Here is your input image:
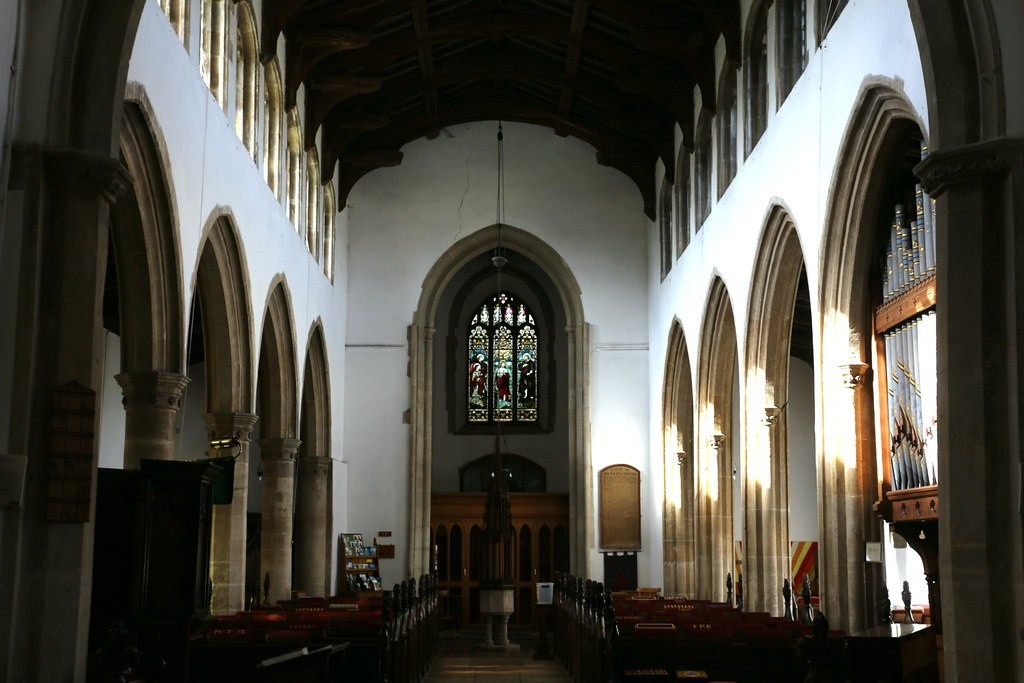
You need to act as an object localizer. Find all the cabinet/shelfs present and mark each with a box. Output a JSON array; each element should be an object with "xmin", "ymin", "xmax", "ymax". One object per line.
[{"xmin": 340, "ymin": 533, "xmax": 382, "ymax": 599}]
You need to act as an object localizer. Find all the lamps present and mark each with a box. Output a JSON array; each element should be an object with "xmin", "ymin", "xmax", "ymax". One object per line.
[
  {"xmin": 465, "ymin": 116, "xmax": 540, "ymax": 422},
  {"xmin": 207, "ymin": 430, "xmax": 242, "ymax": 458}
]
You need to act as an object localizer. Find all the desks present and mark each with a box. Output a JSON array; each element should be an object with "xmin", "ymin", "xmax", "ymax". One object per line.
[{"xmin": 846, "ymin": 623, "xmax": 938, "ymax": 683}]
